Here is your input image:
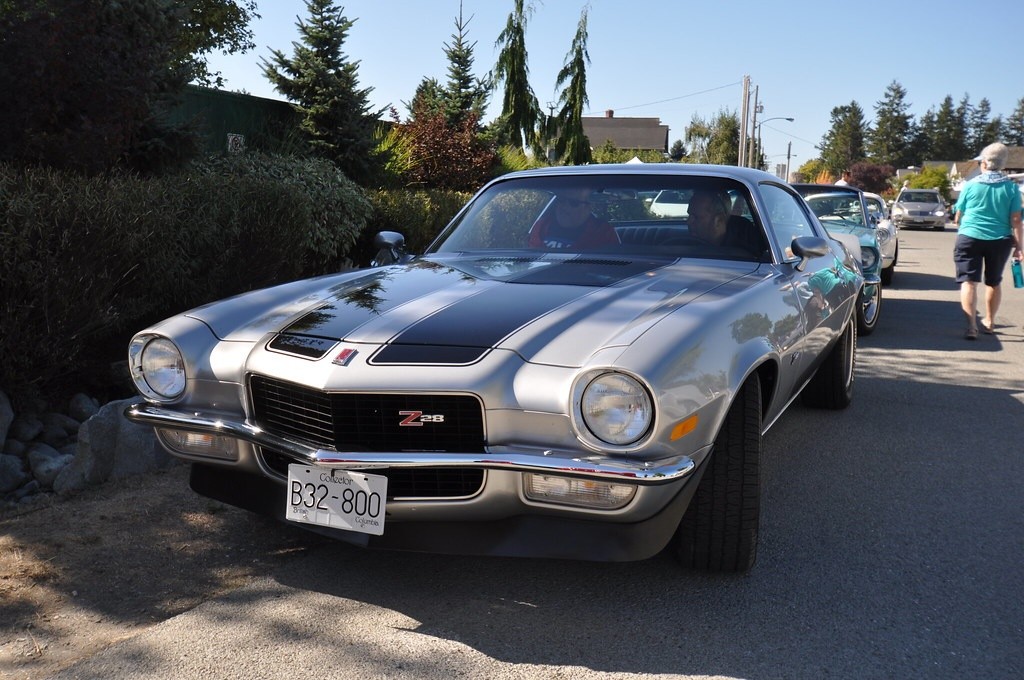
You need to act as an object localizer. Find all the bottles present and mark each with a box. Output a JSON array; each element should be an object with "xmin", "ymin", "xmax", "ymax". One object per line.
[{"xmin": 1011, "ymin": 256, "xmax": 1024, "ymax": 288}]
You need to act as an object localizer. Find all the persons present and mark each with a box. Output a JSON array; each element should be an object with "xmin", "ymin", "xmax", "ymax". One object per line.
[
  {"xmin": 954, "ymin": 142, "xmax": 1024, "ymax": 338},
  {"xmin": 901, "ymin": 179, "xmax": 911, "ymax": 191},
  {"xmin": 686, "ymin": 188, "xmax": 755, "ymax": 253},
  {"xmin": 526, "ymin": 184, "xmax": 622, "ymax": 250},
  {"xmin": 834, "ymin": 171, "xmax": 852, "ymax": 186},
  {"xmin": 803, "ymin": 256, "xmax": 866, "ymax": 319}
]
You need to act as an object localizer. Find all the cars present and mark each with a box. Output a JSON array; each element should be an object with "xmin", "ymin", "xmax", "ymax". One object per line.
[
  {"xmin": 726, "ymin": 182, "xmax": 884, "ymax": 337},
  {"xmin": 646, "ymin": 190, "xmax": 691, "ymax": 221},
  {"xmin": 121, "ymin": 161, "xmax": 870, "ymax": 579},
  {"xmin": 804, "ymin": 191, "xmax": 900, "ymax": 287},
  {"xmin": 888, "ymin": 188, "xmax": 950, "ymax": 231}
]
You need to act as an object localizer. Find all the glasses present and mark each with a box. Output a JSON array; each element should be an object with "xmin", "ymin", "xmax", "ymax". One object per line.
[{"xmin": 556, "ymin": 194, "xmax": 592, "ymax": 208}]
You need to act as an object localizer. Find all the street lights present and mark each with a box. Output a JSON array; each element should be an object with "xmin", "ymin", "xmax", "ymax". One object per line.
[{"xmin": 748, "ymin": 84, "xmax": 795, "ymax": 170}]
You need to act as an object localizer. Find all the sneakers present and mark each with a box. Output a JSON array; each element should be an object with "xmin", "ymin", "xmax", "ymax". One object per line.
[
  {"xmin": 965, "ymin": 327, "xmax": 979, "ymax": 339},
  {"xmin": 978, "ymin": 319, "xmax": 993, "ymax": 334}
]
